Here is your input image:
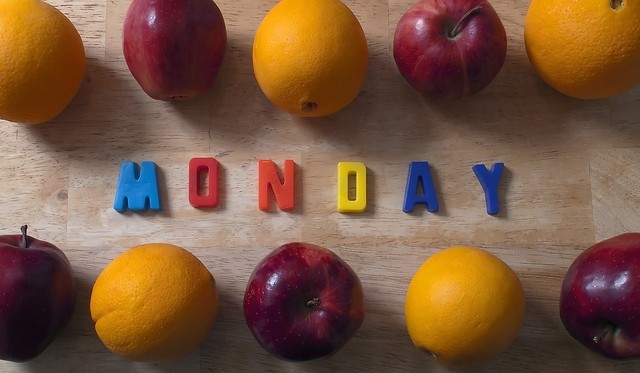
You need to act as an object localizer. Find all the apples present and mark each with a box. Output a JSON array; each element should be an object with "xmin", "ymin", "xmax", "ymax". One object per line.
[
  {"xmin": 242, "ymin": 241, "xmax": 365, "ymax": 361},
  {"xmin": 122, "ymin": 1, "xmax": 226, "ymax": 103},
  {"xmin": 559, "ymin": 232, "xmax": 640, "ymax": 361},
  {"xmin": 1, "ymin": 225, "xmax": 76, "ymax": 363},
  {"xmin": 393, "ymin": 0, "xmax": 508, "ymax": 100}
]
